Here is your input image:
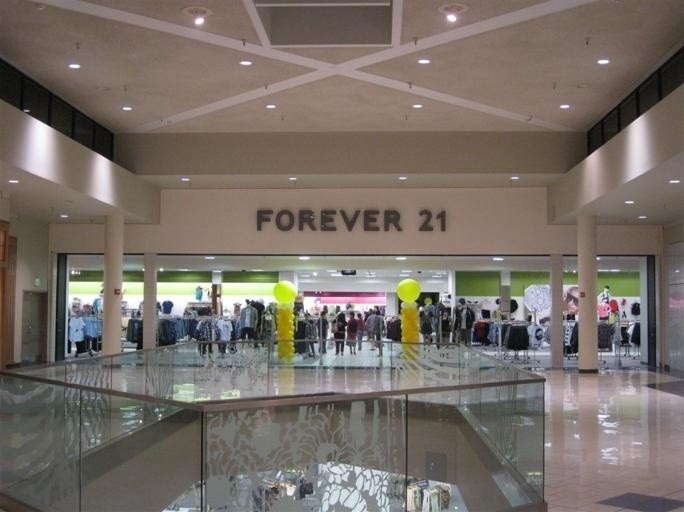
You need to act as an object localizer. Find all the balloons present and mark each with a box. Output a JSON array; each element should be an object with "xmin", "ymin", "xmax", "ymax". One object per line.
[
  {"xmin": 397, "ymin": 278, "xmax": 421, "ymax": 357},
  {"xmin": 273, "ymin": 279, "xmax": 297, "ymax": 360}
]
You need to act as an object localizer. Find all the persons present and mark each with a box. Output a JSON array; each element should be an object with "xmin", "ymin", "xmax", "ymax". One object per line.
[
  {"xmin": 238, "ymin": 298, "xmax": 260, "ymax": 351},
  {"xmin": 416, "ymin": 294, "xmax": 476, "ymax": 352},
  {"xmin": 563, "ymin": 286, "xmax": 580, "ymax": 315},
  {"xmin": 596, "ymin": 298, "xmax": 612, "ymax": 322},
  {"xmin": 597, "ymin": 285, "xmax": 613, "ymax": 304},
  {"xmin": 294, "ymin": 304, "xmax": 384, "ymax": 359}
]
